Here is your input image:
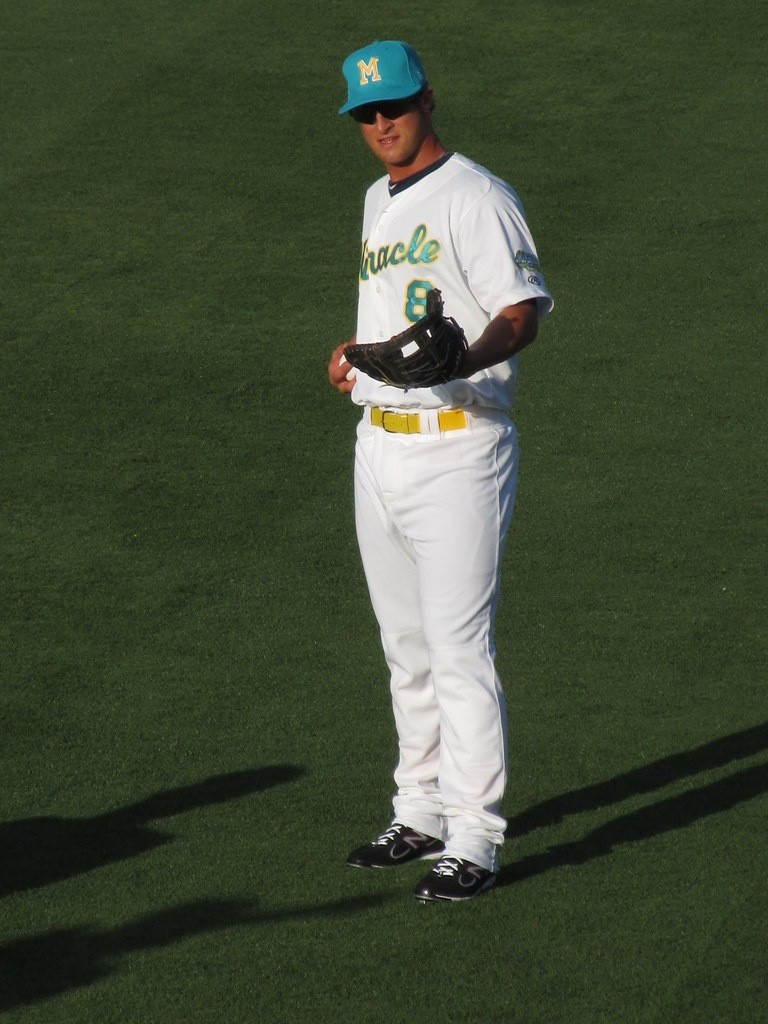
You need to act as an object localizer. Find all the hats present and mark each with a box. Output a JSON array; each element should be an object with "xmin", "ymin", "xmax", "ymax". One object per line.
[{"xmin": 338, "ymin": 39, "xmax": 427, "ymax": 115}]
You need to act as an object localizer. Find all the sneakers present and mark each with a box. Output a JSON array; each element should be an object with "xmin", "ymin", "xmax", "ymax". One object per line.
[
  {"xmin": 344, "ymin": 818, "xmax": 441, "ymax": 870},
  {"xmin": 414, "ymin": 845, "xmax": 498, "ymax": 900}
]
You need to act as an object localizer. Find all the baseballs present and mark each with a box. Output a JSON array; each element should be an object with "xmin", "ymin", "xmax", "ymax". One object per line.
[{"xmin": 337, "ymin": 353, "xmax": 358, "ymax": 383}]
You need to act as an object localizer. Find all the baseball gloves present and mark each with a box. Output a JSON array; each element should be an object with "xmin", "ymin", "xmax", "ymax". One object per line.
[{"xmin": 342, "ymin": 288, "xmax": 471, "ymax": 394}]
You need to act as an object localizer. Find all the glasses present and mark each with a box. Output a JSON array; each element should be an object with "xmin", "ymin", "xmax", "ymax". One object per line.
[{"xmin": 349, "ymin": 97, "xmax": 420, "ymax": 124}]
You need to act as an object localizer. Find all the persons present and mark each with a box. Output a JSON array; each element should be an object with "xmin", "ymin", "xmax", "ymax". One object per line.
[{"xmin": 327, "ymin": 39, "xmax": 556, "ymax": 907}]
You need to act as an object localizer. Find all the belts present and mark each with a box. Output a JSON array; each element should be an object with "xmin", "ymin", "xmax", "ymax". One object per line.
[{"xmin": 372, "ymin": 407, "xmax": 466, "ymax": 432}]
